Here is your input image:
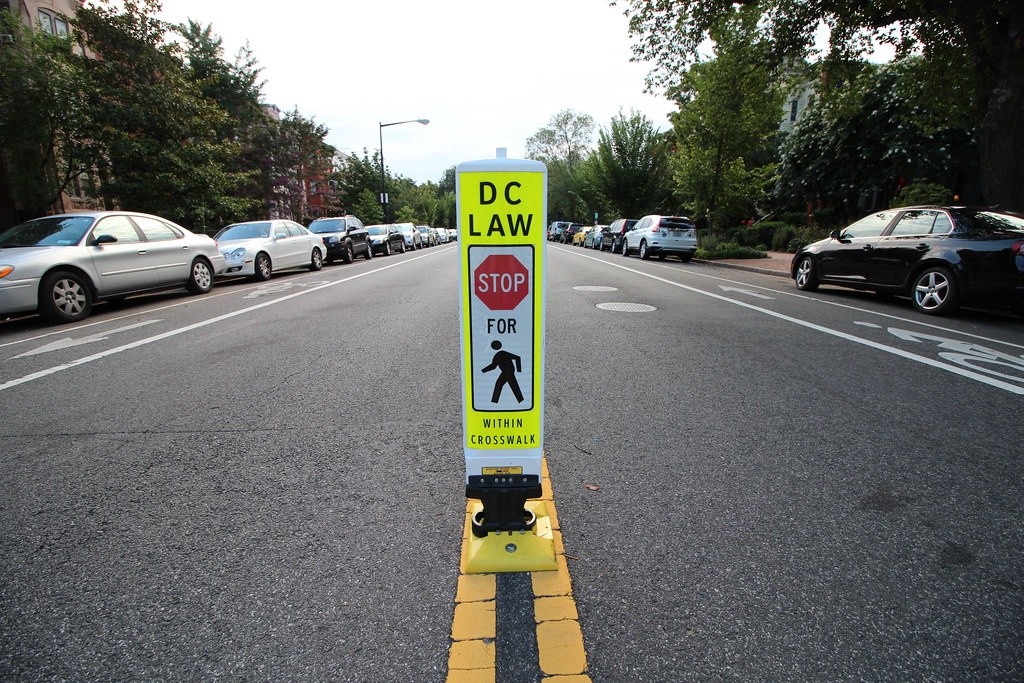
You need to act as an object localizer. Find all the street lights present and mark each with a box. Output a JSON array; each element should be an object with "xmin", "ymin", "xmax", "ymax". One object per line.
[{"xmin": 378, "ymin": 118, "xmax": 430, "ymax": 223}]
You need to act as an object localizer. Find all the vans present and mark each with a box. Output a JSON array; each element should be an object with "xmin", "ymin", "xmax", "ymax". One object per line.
[{"xmin": 550, "ymin": 220, "xmax": 571, "ymax": 240}]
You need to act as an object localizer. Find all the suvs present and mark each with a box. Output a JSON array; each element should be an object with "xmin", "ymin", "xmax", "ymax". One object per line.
[
  {"xmin": 600, "ymin": 219, "xmax": 639, "ymax": 254},
  {"xmin": 621, "ymin": 214, "xmax": 699, "ymax": 263},
  {"xmin": 307, "ymin": 215, "xmax": 374, "ymax": 262},
  {"xmin": 559, "ymin": 222, "xmax": 580, "ymax": 243}
]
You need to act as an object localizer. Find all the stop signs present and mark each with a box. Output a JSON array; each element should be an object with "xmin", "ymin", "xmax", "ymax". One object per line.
[{"xmin": 459, "ymin": 170, "xmax": 540, "ymax": 478}]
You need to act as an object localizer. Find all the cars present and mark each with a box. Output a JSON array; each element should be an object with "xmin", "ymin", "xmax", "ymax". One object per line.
[
  {"xmin": 584, "ymin": 225, "xmax": 609, "ymax": 249},
  {"xmin": 790, "ymin": 204, "xmax": 1024, "ymax": 323},
  {"xmin": 547, "ymin": 225, "xmax": 551, "ymax": 238},
  {"xmin": 572, "ymin": 226, "xmax": 593, "ymax": 247},
  {"xmin": 1, "ymin": 208, "xmax": 226, "ymax": 323},
  {"xmin": 365, "ymin": 225, "xmax": 406, "ymax": 254},
  {"xmin": 213, "ymin": 218, "xmax": 328, "ymax": 280},
  {"xmin": 393, "ymin": 222, "xmax": 458, "ymax": 251}
]
